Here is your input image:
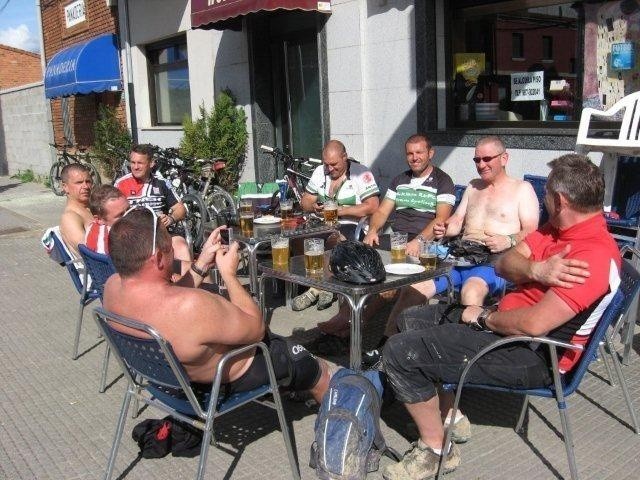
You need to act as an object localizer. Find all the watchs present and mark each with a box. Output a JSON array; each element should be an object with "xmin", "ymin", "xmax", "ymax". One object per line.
[
  {"xmin": 477, "ymin": 309, "xmax": 493, "ymax": 334},
  {"xmin": 508, "ymin": 235, "xmax": 517, "ymax": 249}
]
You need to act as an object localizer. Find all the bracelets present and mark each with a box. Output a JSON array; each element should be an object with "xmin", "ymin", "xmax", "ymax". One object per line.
[
  {"xmin": 414, "ymin": 235, "xmax": 426, "ymax": 243},
  {"xmin": 189, "ymin": 263, "xmax": 209, "ymax": 279},
  {"xmin": 170, "ymin": 216, "xmax": 177, "ymax": 222}
]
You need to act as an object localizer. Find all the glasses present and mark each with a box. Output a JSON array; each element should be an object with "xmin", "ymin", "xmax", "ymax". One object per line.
[
  {"xmin": 116, "ymin": 202, "xmax": 161, "ymax": 257},
  {"xmin": 472, "ymin": 152, "xmax": 505, "ymax": 163}
]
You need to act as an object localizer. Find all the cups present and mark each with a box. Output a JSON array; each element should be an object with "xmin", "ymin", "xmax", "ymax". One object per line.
[
  {"xmin": 271, "ymin": 237, "xmax": 289, "ymax": 265},
  {"xmin": 216, "ymin": 200, "xmax": 339, "ymax": 233},
  {"xmin": 419, "ymin": 241, "xmax": 437, "ymax": 272},
  {"xmin": 390, "ymin": 231, "xmax": 408, "ymax": 260},
  {"xmin": 304, "ymin": 238, "xmax": 323, "ymax": 273}
]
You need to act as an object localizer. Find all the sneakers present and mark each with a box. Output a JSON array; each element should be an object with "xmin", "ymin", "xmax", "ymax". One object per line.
[
  {"xmin": 380, "ymin": 434, "xmax": 463, "ymax": 479},
  {"xmin": 291, "ymin": 290, "xmax": 316, "ymax": 311},
  {"xmin": 316, "ymin": 290, "xmax": 335, "ymax": 311},
  {"xmin": 443, "ymin": 415, "xmax": 475, "ymax": 444}
]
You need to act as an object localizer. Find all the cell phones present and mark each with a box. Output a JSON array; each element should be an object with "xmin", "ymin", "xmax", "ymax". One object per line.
[{"xmin": 220, "ymin": 228, "xmax": 233, "ymax": 253}]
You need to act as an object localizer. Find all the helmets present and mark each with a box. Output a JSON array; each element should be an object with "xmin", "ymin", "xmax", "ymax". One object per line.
[{"xmin": 328, "ymin": 239, "xmax": 388, "ymax": 286}]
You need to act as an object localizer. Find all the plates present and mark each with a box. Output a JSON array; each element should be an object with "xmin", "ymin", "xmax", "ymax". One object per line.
[{"xmin": 383, "ymin": 263, "xmax": 424, "ymax": 276}]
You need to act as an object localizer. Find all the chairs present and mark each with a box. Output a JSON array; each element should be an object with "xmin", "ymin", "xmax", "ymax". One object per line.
[{"xmin": 50, "ymin": 194, "xmax": 640, "ymax": 480}]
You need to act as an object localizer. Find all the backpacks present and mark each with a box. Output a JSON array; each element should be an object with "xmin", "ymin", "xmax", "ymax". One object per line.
[{"xmin": 307, "ymin": 366, "xmax": 391, "ymax": 480}]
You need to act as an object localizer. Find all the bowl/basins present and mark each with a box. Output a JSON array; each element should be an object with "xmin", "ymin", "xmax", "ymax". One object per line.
[{"xmin": 477, "ymin": 103, "xmax": 499, "ymax": 120}]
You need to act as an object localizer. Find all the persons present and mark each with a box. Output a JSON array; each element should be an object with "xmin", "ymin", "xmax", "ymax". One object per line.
[
  {"xmin": 60, "ymin": 164, "xmax": 191, "ymax": 261},
  {"xmin": 103, "ymin": 206, "xmax": 330, "ymax": 407},
  {"xmin": 84, "ymin": 185, "xmax": 193, "ymax": 282},
  {"xmin": 317, "ymin": 134, "xmax": 456, "ymax": 339},
  {"xmin": 382, "ymin": 153, "xmax": 621, "ymax": 479},
  {"xmin": 291, "ymin": 140, "xmax": 381, "ymax": 311},
  {"xmin": 113, "ymin": 145, "xmax": 187, "ymax": 228},
  {"xmin": 377, "ymin": 135, "xmax": 540, "ymax": 358}
]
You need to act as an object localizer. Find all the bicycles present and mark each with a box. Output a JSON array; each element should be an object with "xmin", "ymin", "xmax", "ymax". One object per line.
[
  {"xmin": 103, "ymin": 141, "xmax": 237, "ymax": 252},
  {"xmin": 259, "ymin": 142, "xmax": 325, "ymax": 213},
  {"xmin": 47, "ymin": 141, "xmax": 102, "ymax": 194}
]
